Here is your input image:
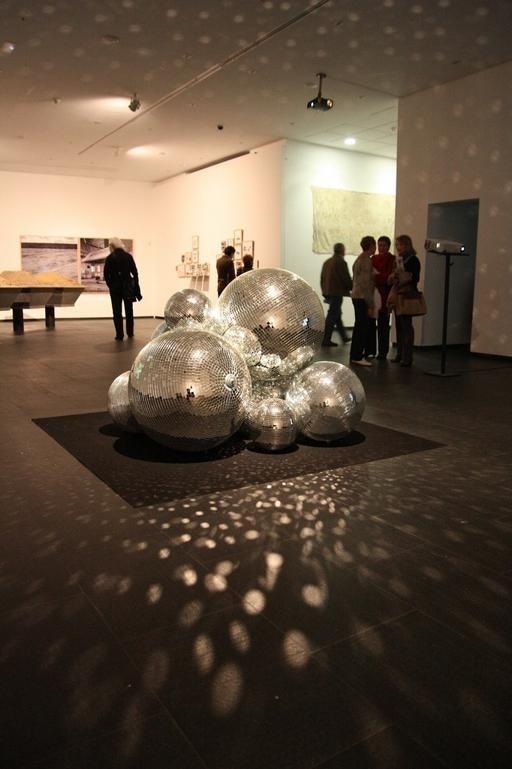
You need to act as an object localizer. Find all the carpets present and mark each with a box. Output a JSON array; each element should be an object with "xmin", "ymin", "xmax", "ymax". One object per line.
[{"xmin": 32, "ymin": 411, "xmax": 447, "ymax": 508}]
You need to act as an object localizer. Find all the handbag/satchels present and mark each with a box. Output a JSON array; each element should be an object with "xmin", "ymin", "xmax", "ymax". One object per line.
[{"xmin": 395, "ymin": 291, "xmax": 427, "ymax": 316}]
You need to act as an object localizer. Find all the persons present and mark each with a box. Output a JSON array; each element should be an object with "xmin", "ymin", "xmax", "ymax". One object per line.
[
  {"xmin": 237, "ymin": 254, "xmax": 254, "ymax": 278},
  {"xmin": 386, "ymin": 234, "xmax": 421, "ymax": 367},
  {"xmin": 349, "ymin": 236, "xmax": 376, "ymax": 366},
  {"xmin": 320, "ymin": 243, "xmax": 353, "ymax": 347},
  {"xmin": 103, "ymin": 237, "xmax": 142, "ymax": 340},
  {"xmin": 216, "ymin": 246, "xmax": 235, "ymax": 297},
  {"xmin": 370, "ymin": 236, "xmax": 396, "ymax": 359}
]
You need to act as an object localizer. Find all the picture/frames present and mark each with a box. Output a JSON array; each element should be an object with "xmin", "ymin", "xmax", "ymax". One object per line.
[
  {"xmin": 216, "ymin": 229, "xmax": 259, "ymax": 274},
  {"xmin": 175, "ymin": 235, "xmax": 210, "ymax": 277}
]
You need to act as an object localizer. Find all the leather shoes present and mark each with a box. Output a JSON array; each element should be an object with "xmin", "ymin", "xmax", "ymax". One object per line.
[
  {"xmin": 322, "ymin": 341, "xmax": 337, "ymax": 346},
  {"xmin": 351, "ymin": 357, "xmax": 372, "ymax": 366},
  {"xmin": 400, "ymin": 359, "xmax": 411, "ymax": 367},
  {"xmin": 391, "ymin": 358, "xmax": 400, "ymax": 362}
]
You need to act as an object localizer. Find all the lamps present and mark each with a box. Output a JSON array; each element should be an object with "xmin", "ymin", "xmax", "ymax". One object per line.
[{"xmin": 128, "ymin": 94, "xmax": 140, "ymax": 112}]
